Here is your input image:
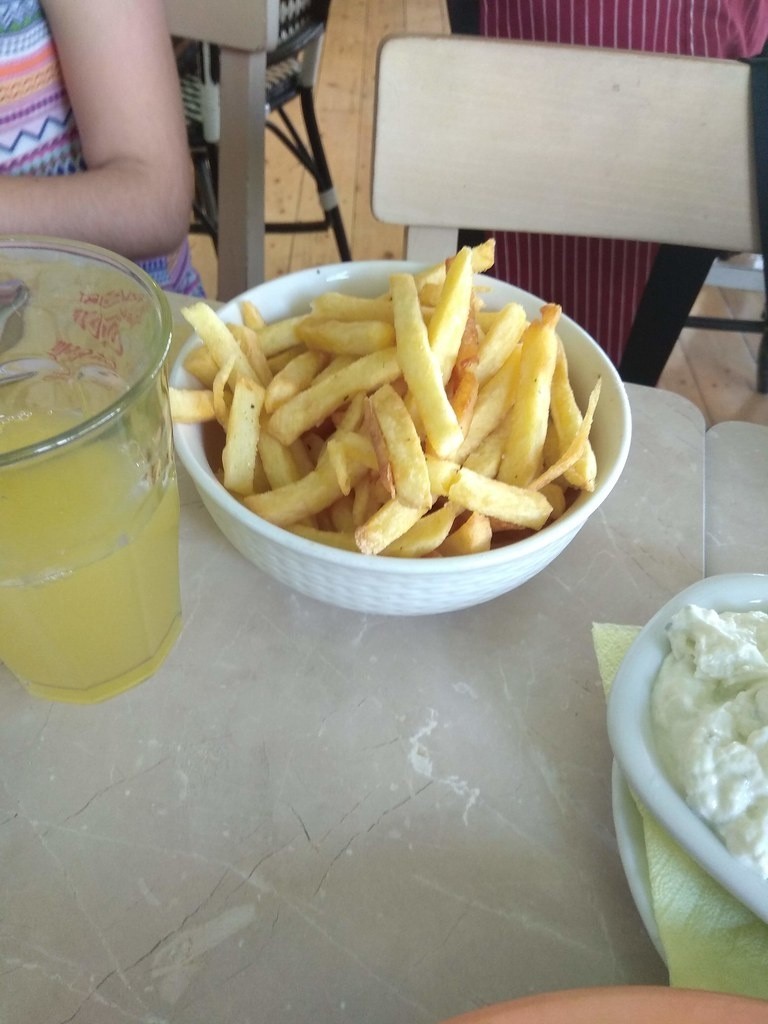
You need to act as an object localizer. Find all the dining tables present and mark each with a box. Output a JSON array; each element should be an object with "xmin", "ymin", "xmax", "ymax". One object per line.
[{"xmin": 0, "ymin": 384, "xmax": 768, "ymax": 1024}]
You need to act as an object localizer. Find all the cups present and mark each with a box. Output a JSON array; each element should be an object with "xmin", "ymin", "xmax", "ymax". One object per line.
[{"xmin": 0, "ymin": 233, "xmax": 181, "ymax": 705}]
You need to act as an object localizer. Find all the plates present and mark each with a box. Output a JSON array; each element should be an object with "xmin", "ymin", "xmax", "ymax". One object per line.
[{"xmin": 607, "ymin": 572, "xmax": 768, "ymax": 965}]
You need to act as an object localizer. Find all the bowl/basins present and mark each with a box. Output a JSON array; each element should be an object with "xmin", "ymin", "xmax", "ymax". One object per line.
[{"xmin": 168, "ymin": 259, "xmax": 633, "ymax": 616}]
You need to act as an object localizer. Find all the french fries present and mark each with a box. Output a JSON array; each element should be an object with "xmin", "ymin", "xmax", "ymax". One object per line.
[{"xmin": 167, "ymin": 240, "xmax": 598, "ymax": 558}]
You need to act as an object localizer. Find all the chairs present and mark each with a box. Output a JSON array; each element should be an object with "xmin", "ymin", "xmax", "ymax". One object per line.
[{"xmin": 163, "ymin": 0, "xmax": 768, "ymax": 395}]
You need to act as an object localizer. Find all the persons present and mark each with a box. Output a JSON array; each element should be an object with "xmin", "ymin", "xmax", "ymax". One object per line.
[
  {"xmin": 446, "ymin": 0, "xmax": 767, "ymax": 387},
  {"xmin": 0, "ymin": 0, "xmax": 206, "ymax": 301}
]
